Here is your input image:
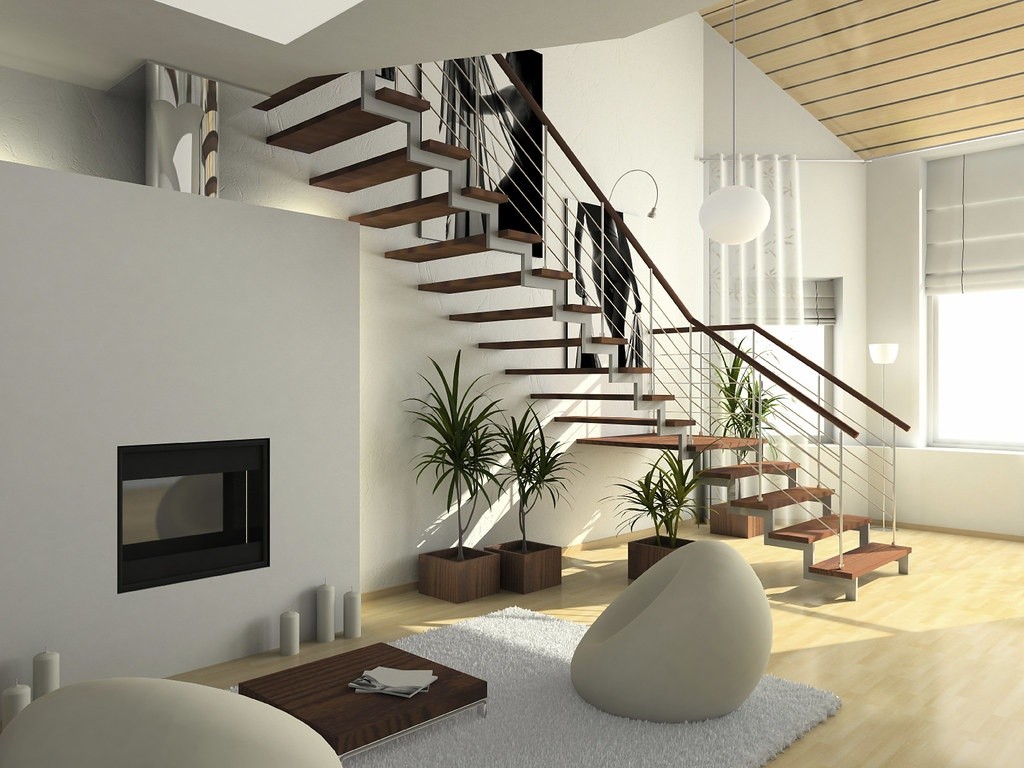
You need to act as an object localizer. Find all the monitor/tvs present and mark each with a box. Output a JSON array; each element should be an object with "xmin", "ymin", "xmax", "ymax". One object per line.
[{"xmin": 120, "ymin": 470, "xmax": 249, "ymax": 561}]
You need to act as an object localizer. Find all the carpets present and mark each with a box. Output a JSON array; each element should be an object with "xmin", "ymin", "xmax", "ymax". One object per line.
[{"xmin": 225, "ymin": 606, "xmax": 841, "ymax": 768}]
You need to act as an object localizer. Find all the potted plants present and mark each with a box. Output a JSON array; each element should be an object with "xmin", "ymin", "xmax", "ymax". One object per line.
[
  {"xmin": 409, "ymin": 351, "xmax": 500, "ymax": 604},
  {"xmin": 709, "ymin": 333, "xmax": 787, "ymax": 540},
  {"xmin": 482, "ymin": 396, "xmax": 586, "ymax": 594},
  {"xmin": 611, "ymin": 439, "xmax": 718, "ymax": 581}
]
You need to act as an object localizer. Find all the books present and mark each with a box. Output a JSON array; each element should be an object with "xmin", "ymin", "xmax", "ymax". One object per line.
[{"xmin": 348, "ymin": 666, "xmax": 438, "ymax": 698}]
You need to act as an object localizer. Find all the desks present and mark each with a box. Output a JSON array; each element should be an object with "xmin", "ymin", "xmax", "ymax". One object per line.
[{"xmin": 238, "ymin": 641, "xmax": 488, "ymax": 759}]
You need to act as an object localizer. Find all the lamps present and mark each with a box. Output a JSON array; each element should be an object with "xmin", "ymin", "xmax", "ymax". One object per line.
[
  {"xmin": 608, "ymin": 168, "xmax": 660, "ymax": 218},
  {"xmin": 698, "ymin": 0, "xmax": 770, "ymax": 247},
  {"xmin": 869, "ymin": 342, "xmax": 899, "ymax": 530}
]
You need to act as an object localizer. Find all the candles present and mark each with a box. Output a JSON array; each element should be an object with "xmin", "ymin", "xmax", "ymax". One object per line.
[
  {"xmin": 316, "ymin": 578, "xmax": 335, "ymax": 643},
  {"xmin": 33, "ymin": 646, "xmax": 60, "ymax": 702},
  {"xmin": 343, "ymin": 586, "xmax": 361, "ymax": 639},
  {"xmin": 3, "ymin": 677, "xmax": 33, "ymax": 733},
  {"xmin": 280, "ymin": 609, "xmax": 300, "ymax": 658}
]
